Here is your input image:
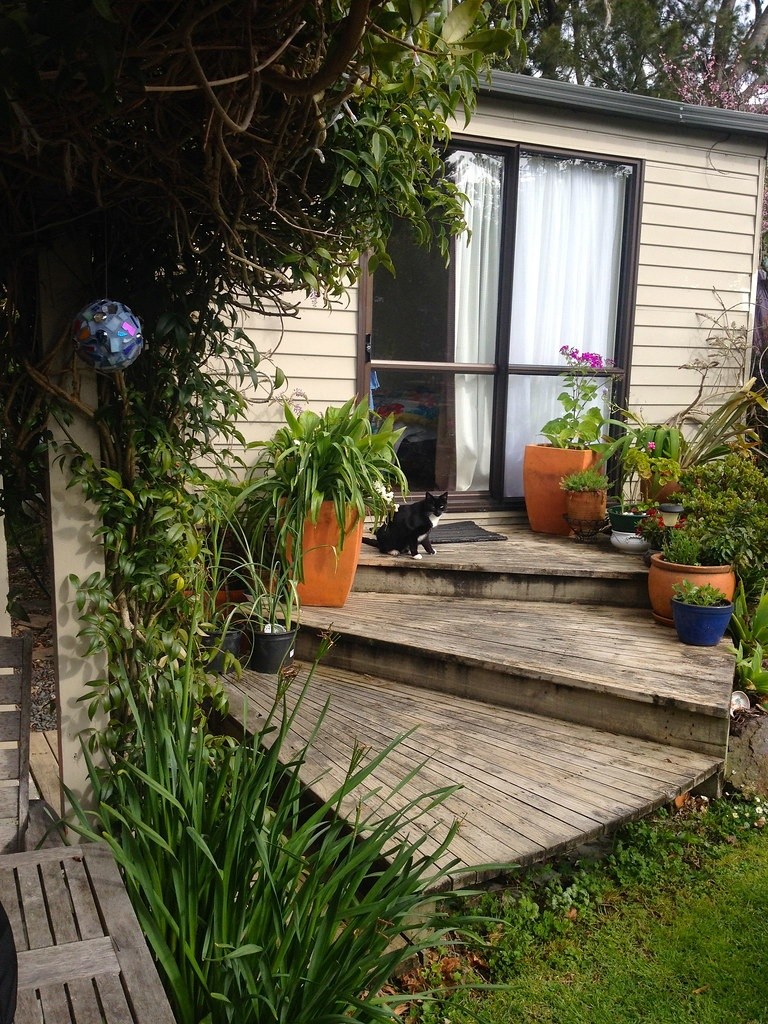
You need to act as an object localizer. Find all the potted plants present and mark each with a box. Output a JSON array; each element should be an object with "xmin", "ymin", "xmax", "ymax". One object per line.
[
  {"xmin": 201, "ymin": 488, "xmax": 246, "ymax": 676},
  {"xmin": 668, "ymin": 576, "xmax": 734, "ymax": 646},
  {"xmin": 559, "ymin": 464, "xmax": 619, "ymax": 522},
  {"xmin": 204, "ymin": 488, "xmax": 340, "ymax": 675},
  {"xmin": 645, "ymin": 527, "xmax": 738, "ymax": 629},
  {"xmin": 635, "ymin": 426, "xmax": 694, "ymax": 503},
  {"xmin": 249, "ymin": 388, "xmax": 411, "ymax": 610}
]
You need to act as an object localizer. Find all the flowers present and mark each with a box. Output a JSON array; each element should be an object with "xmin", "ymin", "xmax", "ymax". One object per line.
[
  {"xmin": 534, "ymin": 344, "xmax": 627, "ymax": 450},
  {"xmin": 608, "ymin": 438, "xmax": 680, "ymax": 513}
]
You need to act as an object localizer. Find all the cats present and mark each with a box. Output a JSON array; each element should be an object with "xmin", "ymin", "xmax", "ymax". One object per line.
[{"xmin": 361, "ymin": 491, "xmax": 449, "ymax": 561}]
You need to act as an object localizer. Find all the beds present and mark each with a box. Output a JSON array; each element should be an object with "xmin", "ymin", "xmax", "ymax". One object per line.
[{"xmin": 377, "ymin": 384, "xmax": 442, "ymax": 486}]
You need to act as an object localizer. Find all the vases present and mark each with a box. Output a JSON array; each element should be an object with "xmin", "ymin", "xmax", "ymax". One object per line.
[
  {"xmin": 524, "ymin": 442, "xmax": 599, "ymax": 535},
  {"xmin": 606, "ymin": 503, "xmax": 655, "ymax": 556}
]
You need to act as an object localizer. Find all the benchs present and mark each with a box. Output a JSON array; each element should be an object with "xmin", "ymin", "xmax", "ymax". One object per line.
[{"xmin": 0, "ymin": 838, "xmax": 177, "ymax": 1024}]
